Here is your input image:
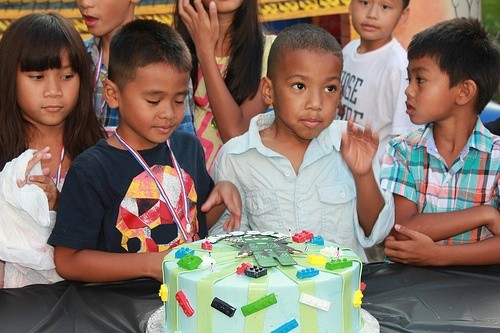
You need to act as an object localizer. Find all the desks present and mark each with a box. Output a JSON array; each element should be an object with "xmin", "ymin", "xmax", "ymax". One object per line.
[{"xmin": 0, "ymin": 262, "xmax": 500, "ymax": 333}]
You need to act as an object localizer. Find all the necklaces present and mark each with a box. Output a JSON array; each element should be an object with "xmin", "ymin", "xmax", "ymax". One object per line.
[
  {"xmin": 55, "ymin": 144, "xmax": 65, "ymax": 190},
  {"xmin": 92, "ymin": 38, "xmax": 107, "ymax": 125},
  {"xmin": 113, "ymin": 128, "xmax": 191, "ymax": 241}
]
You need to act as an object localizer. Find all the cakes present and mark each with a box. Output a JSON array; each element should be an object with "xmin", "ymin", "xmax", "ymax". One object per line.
[{"xmin": 158, "ymin": 230, "xmax": 366, "ymax": 333}]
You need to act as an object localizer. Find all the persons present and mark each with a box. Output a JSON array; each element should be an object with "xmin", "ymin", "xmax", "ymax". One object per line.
[
  {"xmin": 174, "ymin": 1, "xmax": 281, "ymax": 175},
  {"xmin": 47, "ymin": 19, "xmax": 242, "ymax": 283},
  {"xmin": 336, "ymin": 0, "xmax": 426, "ymax": 167},
  {"xmin": 0, "ymin": 12, "xmax": 108, "ymax": 288},
  {"xmin": 75, "ymin": 0, "xmax": 197, "ymax": 138},
  {"xmin": 207, "ymin": 24, "xmax": 395, "ymax": 264},
  {"xmin": 379, "ymin": 17, "xmax": 500, "ymax": 266}
]
